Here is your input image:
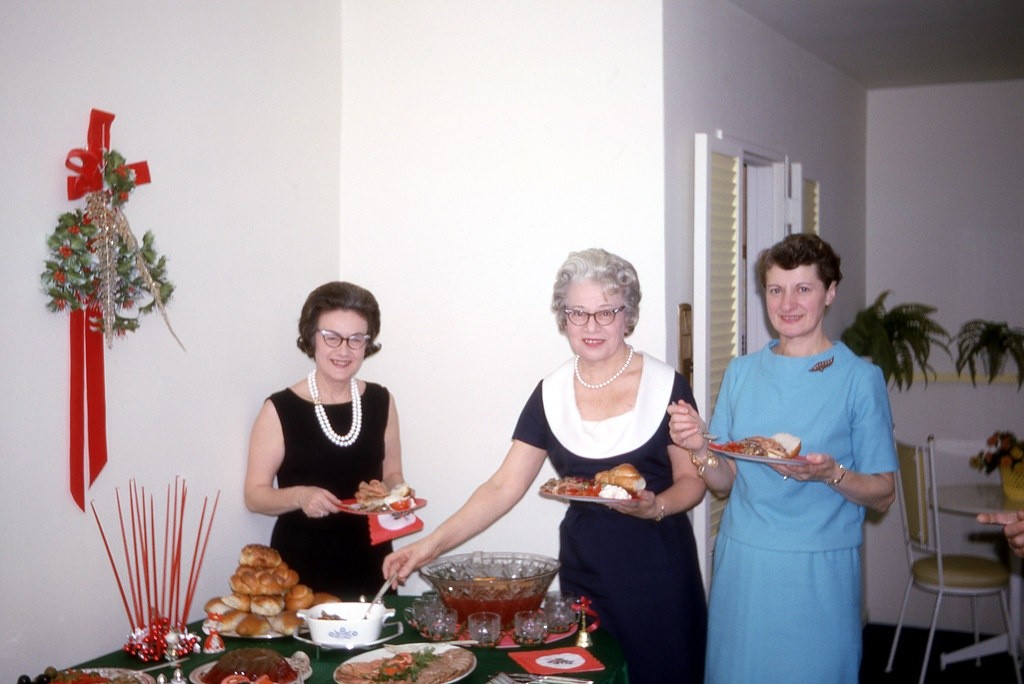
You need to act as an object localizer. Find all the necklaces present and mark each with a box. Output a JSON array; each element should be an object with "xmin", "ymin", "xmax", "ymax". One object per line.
[
  {"xmin": 308, "ymin": 370, "xmax": 362, "ymax": 447},
  {"xmin": 575, "ymin": 346, "xmax": 634, "ymax": 389}
]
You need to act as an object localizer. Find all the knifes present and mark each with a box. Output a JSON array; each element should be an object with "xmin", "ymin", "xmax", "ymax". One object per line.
[{"xmin": 488, "ymin": 672, "xmax": 594, "ymax": 684}]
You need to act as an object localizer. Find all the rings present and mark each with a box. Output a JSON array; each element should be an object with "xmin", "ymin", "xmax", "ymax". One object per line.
[{"xmin": 784, "ymin": 474, "xmax": 789, "ymax": 481}]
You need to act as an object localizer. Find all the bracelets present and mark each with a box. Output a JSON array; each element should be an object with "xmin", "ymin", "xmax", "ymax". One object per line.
[
  {"xmin": 688, "ymin": 432, "xmax": 719, "ymax": 477},
  {"xmin": 825, "ymin": 463, "xmax": 847, "ymax": 487},
  {"xmin": 654, "ymin": 497, "xmax": 666, "ymax": 521}
]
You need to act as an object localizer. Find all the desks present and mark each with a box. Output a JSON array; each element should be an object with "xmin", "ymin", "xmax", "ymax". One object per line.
[
  {"xmin": 76, "ymin": 594, "xmax": 629, "ymax": 684},
  {"xmin": 927, "ymin": 483, "xmax": 1024, "ymax": 674}
]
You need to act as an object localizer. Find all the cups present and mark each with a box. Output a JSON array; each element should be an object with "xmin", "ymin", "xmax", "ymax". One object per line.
[
  {"xmin": 544, "ymin": 591, "xmax": 581, "ymax": 633},
  {"xmin": 403, "ymin": 598, "xmax": 444, "ymax": 629},
  {"xmin": 425, "ymin": 608, "xmax": 457, "ymax": 640},
  {"xmin": 514, "ymin": 611, "xmax": 548, "ymax": 638},
  {"xmin": 468, "ymin": 611, "xmax": 500, "ymax": 641}
]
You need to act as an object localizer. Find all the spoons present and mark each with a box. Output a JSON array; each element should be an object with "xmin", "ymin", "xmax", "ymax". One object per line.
[{"xmin": 289, "ymin": 651, "xmax": 310, "ymax": 684}]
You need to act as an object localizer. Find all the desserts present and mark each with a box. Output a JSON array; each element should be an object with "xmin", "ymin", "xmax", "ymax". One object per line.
[{"xmin": 203, "ymin": 647, "xmax": 297, "ymax": 684}]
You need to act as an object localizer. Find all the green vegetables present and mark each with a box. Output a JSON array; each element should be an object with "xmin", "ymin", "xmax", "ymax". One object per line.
[{"xmin": 370, "ymin": 645, "xmax": 443, "ymax": 682}]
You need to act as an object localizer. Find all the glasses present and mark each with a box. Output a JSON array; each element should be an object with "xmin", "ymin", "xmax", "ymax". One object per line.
[
  {"xmin": 565, "ymin": 306, "xmax": 624, "ymax": 326},
  {"xmin": 317, "ymin": 328, "xmax": 370, "ymax": 349}
]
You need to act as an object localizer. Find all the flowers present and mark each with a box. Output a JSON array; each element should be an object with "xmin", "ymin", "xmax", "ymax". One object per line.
[{"xmin": 969, "ymin": 429, "xmax": 1024, "ymax": 477}]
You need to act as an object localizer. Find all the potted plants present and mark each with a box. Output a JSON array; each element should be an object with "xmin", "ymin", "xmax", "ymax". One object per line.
[{"xmin": 943, "ymin": 319, "xmax": 1024, "ymax": 392}]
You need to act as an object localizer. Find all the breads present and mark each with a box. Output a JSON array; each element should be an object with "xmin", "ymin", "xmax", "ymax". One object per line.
[
  {"xmin": 773, "ymin": 433, "xmax": 801, "ymax": 458},
  {"xmin": 390, "ymin": 483, "xmax": 412, "ymax": 497},
  {"xmin": 205, "ymin": 545, "xmax": 340, "ymax": 636},
  {"xmin": 595, "ymin": 464, "xmax": 647, "ymax": 492}
]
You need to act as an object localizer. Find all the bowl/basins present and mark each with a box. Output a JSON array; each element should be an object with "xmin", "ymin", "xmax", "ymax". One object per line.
[
  {"xmin": 418, "ymin": 551, "xmax": 561, "ymax": 630},
  {"xmin": 295, "ymin": 601, "xmax": 396, "ymax": 648}
]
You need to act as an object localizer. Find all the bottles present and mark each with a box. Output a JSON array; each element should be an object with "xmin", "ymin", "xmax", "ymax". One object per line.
[
  {"xmin": 170, "ymin": 668, "xmax": 188, "ymax": 684},
  {"xmin": 157, "ymin": 673, "xmax": 170, "ymax": 684}
]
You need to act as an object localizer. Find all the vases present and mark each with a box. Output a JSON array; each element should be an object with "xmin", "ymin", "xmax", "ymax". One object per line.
[{"xmin": 998, "ymin": 464, "xmax": 1024, "ymax": 502}]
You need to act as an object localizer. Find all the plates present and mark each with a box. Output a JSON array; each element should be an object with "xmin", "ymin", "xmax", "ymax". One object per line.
[
  {"xmin": 64, "ymin": 666, "xmax": 156, "ymax": 684},
  {"xmin": 708, "ymin": 444, "xmax": 809, "ymax": 466},
  {"xmin": 335, "ymin": 499, "xmax": 427, "ymax": 514},
  {"xmin": 333, "ymin": 643, "xmax": 477, "ymax": 684},
  {"xmin": 539, "ymin": 486, "xmax": 640, "ymax": 502},
  {"xmin": 189, "ymin": 657, "xmax": 313, "ymax": 684}
]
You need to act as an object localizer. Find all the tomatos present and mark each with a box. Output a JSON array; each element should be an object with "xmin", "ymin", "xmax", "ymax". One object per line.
[{"xmin": 221, "ymin": 673, "xmax": 281, "ymax": 684}]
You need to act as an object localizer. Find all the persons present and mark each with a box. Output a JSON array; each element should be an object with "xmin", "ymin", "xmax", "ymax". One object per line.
[
  {"xmin": 667, "ymin": 233, "xmax": 897, "ymax": 684},
  {"xmin": 381, "ymin": 247, "xmax": 707, "ymax": 683},
  {"xmin": 976, "ymin": 510, "xmax": 1024, "ymax": 558},
  {"xmin": 243, "ymin": 281, "xmax": 408, "ymax": 604}
]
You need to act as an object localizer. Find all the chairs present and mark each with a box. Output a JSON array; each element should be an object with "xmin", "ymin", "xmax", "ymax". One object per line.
[{"xmin": 885, "ymin": 422, "xmax": 1023, "ymax": 684}]
